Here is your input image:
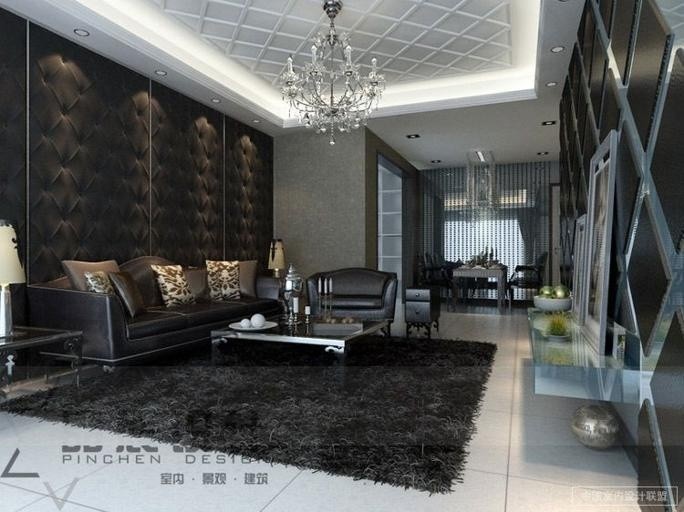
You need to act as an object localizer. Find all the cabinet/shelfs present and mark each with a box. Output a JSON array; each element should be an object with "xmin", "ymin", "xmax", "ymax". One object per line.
[{"xmin": 404, "ymin": 284, "xmax": 441, "ymax": 342}]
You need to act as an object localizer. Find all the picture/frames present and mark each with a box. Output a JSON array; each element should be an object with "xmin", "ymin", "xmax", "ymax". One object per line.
[
  {"xmin": 584, "ymin": 127, "xmax": 621, "ymax": 356},
  {"xmin": 569, "ymin": 213, "xmax": 587, "ymax": 325}
]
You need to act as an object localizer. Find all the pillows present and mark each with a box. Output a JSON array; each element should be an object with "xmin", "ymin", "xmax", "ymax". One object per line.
[
  {"xmin": 107, "ymin": 271, "xmax": 146, "ymax": 321},
  {"xmin": 85, "ymin": 269, "xmax": 120, "ymax": 300},
  {"xmin": 203, "ymin": 257, "xmax": 240, "ymax": 302},
  {"xmin": 232, "ymin": 259, "xmax": 261, "ymax": 300},
  {"xmin": 150, "ymin": 261, "xmax": 198, "ymax": 308},
  {"xmin": 62, "ymin": 255, "xmax": 121, "ymax": 294}
]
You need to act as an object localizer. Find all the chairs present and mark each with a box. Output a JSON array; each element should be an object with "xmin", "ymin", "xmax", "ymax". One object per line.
[{"xmin": 413, "ymin": 247, "xmax": 549, "ymax": 316}]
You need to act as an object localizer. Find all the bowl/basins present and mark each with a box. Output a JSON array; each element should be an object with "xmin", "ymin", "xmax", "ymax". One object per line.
[
  {"xmin": 534, "ymin": 295, "xmax": 571, "ymax": 313},
  {"xmin": 533, "ymin": 316, "xmax": 548, "ymax": 330}
]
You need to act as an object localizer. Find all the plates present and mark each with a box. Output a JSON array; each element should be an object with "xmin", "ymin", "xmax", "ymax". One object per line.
[
  {"xmin": 228, "ymin": 321, "xmax": 278, "ymax": 332},
  {"xmin": 545, "ymin": 334, "xmax": 571, "ymax": 341}
]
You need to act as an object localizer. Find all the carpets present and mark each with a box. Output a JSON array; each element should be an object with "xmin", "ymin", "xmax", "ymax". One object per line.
[{"xmin": 0, "ymin": 333, "xmax": 500, "ymax": 497}]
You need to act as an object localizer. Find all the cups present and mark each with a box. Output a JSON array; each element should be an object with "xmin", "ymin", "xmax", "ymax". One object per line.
[{"xmin": 277, "ymin": 263, "xmax": 304, "ymax": 324}]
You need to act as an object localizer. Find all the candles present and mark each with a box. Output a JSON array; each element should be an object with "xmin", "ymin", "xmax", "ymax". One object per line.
[{"xmin": 318, "ymin": 277, "xmax": 333, "ymax": 294}]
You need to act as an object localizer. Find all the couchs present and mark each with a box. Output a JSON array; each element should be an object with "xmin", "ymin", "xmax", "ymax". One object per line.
[
  {"xmin": 306, "ymin": 267, "xmax": 397, "ymax": 338},
  {"xmin": 26, "ymin": 253, "xmax": 279, "ymax": 377}
]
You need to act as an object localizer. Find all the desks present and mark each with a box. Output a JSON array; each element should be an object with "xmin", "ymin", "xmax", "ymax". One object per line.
[{"xmin": 1, "ymin": 323, "xmax": 86, "ymax": 402}]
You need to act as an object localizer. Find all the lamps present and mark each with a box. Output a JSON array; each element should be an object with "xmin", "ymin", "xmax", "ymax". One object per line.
[
  {"xmin": 274, "ymin": 0, "xmax": 387, "ymax": 146},
  {"xmin": 0, "ymin": 216, "xmax": 29, "ymax": 342},
  {"xmin": 267, "ymin": 237, "xmax": 287, "ymax": 281}
]
